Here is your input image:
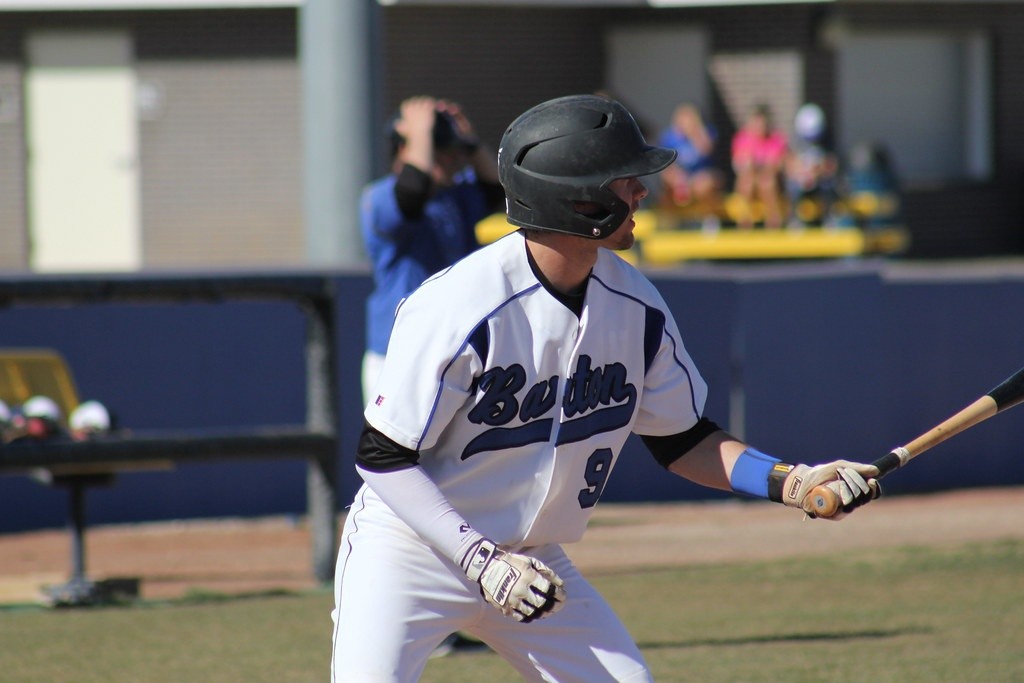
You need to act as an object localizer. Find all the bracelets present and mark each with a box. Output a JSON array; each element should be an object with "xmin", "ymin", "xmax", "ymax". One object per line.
[{"xmin": 729, "ymin": 447, "xmax": 782, "ymax": 500}]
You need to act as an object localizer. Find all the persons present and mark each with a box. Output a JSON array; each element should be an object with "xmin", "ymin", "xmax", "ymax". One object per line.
[
  {"xmin": 362, "ymin": 96, "xmax": 499, "ymax": 412},
  {"xmin": 730, "ymin": 107, "xmax": 789, "ymax": 232},
  {"xmin": 786, "ymin": 103, "xmax": 837, "ymax": 223},
  {"xmin": 329, "ymin": 94, "xmax": 883, "ymax": 682},
  {"xmin": 656, "ymin": 102, "xmax": 724, "ymax": 233}
]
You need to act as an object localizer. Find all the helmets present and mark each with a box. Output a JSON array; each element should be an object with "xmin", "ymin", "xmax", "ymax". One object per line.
[{"xmin": 497, "ymin": 94, "xmax": 678, "ymax": 239}]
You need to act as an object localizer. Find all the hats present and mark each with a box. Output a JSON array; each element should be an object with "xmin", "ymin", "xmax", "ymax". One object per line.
[{"xmin": 387, "ymin": 107, "xmax": 479, "ymax": 154}]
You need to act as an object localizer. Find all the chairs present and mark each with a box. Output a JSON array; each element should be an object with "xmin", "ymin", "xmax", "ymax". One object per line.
[{"xmin": 0, "ymin": 342, "xmax": 179, "ymax": 610}]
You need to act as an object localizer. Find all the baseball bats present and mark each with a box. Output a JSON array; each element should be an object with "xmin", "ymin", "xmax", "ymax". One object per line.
[{"xmin": 809, "ymin": 367, "xmax": 1024, "ymax": 518}]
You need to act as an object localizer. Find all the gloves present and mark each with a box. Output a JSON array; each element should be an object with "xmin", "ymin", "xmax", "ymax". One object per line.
[
  {"xmin": 456, "ymin": 535, "xmax": 568, "ymax": 624},
  {"xmin": 768, "ymin": 458, "xmax": 883, "ymax": 521}
]
